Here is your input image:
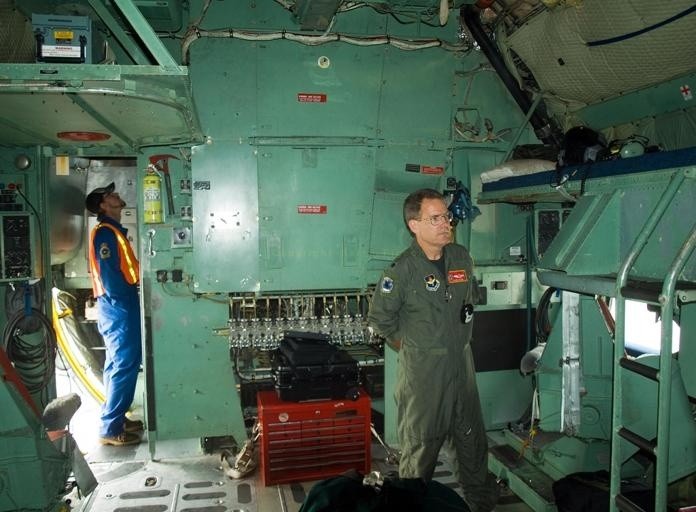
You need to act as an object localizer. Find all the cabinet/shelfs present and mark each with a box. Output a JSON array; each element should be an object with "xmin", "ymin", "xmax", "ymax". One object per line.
[{"xmin": 256, "ymin": 386, "xmax": 371, "ymax": 486}]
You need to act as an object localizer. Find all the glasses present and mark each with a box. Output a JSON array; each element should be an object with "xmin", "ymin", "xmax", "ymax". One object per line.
[{"xmin": 413, "ymin": 210, "xmax": 455, "ymax": 227}]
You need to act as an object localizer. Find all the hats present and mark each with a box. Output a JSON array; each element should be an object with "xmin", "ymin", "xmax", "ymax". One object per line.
[{"xmin": 83, "ymin": 182, "xmax": 117, "ymax": 215}]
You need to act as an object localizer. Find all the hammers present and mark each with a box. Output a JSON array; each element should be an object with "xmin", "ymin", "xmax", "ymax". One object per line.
[{"xmin": 149, "ymin": 155, "xmax": 180, "ymax": 215}]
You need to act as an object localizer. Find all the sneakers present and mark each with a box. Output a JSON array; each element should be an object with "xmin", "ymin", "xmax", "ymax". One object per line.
[
  {"xmin": 98, "ymin": 431, "xmax": 140, "ymax": 448},
  {"xmin": 123, "ymin": 420, "xmax": 145, "ymax": 431}
]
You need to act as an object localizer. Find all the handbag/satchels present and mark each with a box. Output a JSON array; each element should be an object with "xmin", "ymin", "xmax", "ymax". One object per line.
[{"xmin": 280, "ymin": 335, "xmax": 336, "ymax": 364}]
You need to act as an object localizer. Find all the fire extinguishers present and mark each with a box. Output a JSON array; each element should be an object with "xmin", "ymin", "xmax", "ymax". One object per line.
[{"xmin": 143, "ymin": 164, "xmax": 163, "ymax": 224}]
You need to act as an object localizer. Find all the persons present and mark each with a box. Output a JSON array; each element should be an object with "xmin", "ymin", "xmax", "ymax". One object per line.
[
  {"xmin": 82, "ymin": 181, "xmax": 145, "ymax": 447},
  {"xmin": 364, "ymin": 187, "xmax": 503, "ymax": 512}
]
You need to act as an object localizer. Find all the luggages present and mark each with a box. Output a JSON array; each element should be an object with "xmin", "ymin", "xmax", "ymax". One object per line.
[{"xmin": 269, "ymin": 342, "xmax": 361, "ymax": 403}]
